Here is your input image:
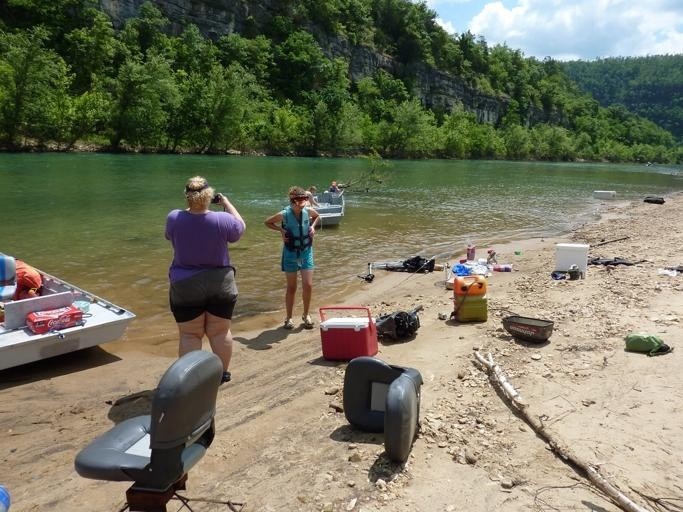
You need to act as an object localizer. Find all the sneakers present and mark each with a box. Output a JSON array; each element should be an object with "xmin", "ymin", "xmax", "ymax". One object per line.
[
  {"xmin": 285, "ymin": 319, "xmax": 294, "ymax": 329},
  {"xmin": 221, "ymin": 370, "xmax": 231, "ymax": 382},
  {"xmin": 303, "ymin": 315, "xmax": 314, "ymax": 328}
]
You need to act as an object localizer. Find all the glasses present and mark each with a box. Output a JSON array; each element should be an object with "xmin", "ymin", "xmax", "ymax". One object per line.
[{"xmin": 290, "ymin": 196, "xmax": 309, "ymax": 203}]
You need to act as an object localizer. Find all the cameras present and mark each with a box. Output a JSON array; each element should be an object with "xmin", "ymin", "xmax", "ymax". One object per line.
[{"xmin": 210, "ymin": 194, "xmax": 220, "ymax": 204}]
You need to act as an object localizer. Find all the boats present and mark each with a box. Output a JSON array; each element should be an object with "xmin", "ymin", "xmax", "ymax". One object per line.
[
  {"xmin": 0, "ymin": 252, "xmax": 136, "ymax": 372},
  {"xmin": 284, "ymin": 190, "xmax": 344, "ymax": 225}
]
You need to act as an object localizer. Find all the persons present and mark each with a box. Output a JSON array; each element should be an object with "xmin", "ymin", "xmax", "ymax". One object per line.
[
  {"xmin": 163, "ymin": 174, "xmax": 246, "ymax": 383},
  {"xmin": 263, "ymin": 185, "xmax": 321, "ymax": 329},
  {"xmin": 303, "ymin": 186, "xmax": 322, "ymax": 208},
  {"xmin": 328, "ymin": 181, "xmax": 342, "ymax": 193}
]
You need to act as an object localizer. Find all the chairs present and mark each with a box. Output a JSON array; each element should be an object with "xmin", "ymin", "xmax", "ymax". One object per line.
[
  {"xmin": 344, "ymin": 356, "xmax": 423, "ymax": 462},
  {"xmin": 74, "ymin": 350, "xmax": 223, "ymax": 512}
]
